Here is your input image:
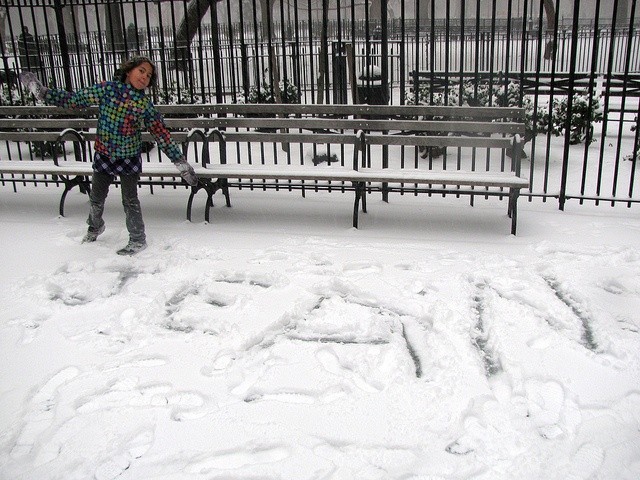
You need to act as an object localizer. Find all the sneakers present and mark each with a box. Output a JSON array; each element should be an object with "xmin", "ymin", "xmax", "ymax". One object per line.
[
  {"xmin": 117, "ymin": 236, "xmax": 148, "ymax": 257},
  {"xmin": 82, "ymin": 225, "xmax": 105, "ymax": 244}
]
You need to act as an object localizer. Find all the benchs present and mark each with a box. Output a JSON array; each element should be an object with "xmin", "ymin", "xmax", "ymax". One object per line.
[
  {"xmin": 409, "ymin": 71, "xmax": 598, "ymax": 119},
  {"xmin": 1, "ymin": 106, "xmax": 526, "ymax": 234},
  {"xmin": 603, "ymin": 73, "xmax": 638, "ymax": 134}
]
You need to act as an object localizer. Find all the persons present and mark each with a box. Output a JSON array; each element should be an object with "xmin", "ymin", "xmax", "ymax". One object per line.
[
  {"xmin": 17, "ymin": 55, "xmax": 199, "ymax": 256},
  {"xmin": 17, "ymin": 23, "xmax": 34, "ymax": 44}
]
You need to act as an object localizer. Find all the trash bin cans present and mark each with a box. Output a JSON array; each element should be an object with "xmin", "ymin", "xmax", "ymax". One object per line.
[{"xmin": 352, "ymin": 65, "xmax": 390, "ymax": 132}]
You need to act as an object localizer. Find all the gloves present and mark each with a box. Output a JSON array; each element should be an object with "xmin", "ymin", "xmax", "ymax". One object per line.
[
  {"xmin": 174, "ymin": 153, "xmax": 198, "ymax": 187},
  {"xmin": 19, "ymin": 71, "xmax": 48, "ymax": 100}
]
